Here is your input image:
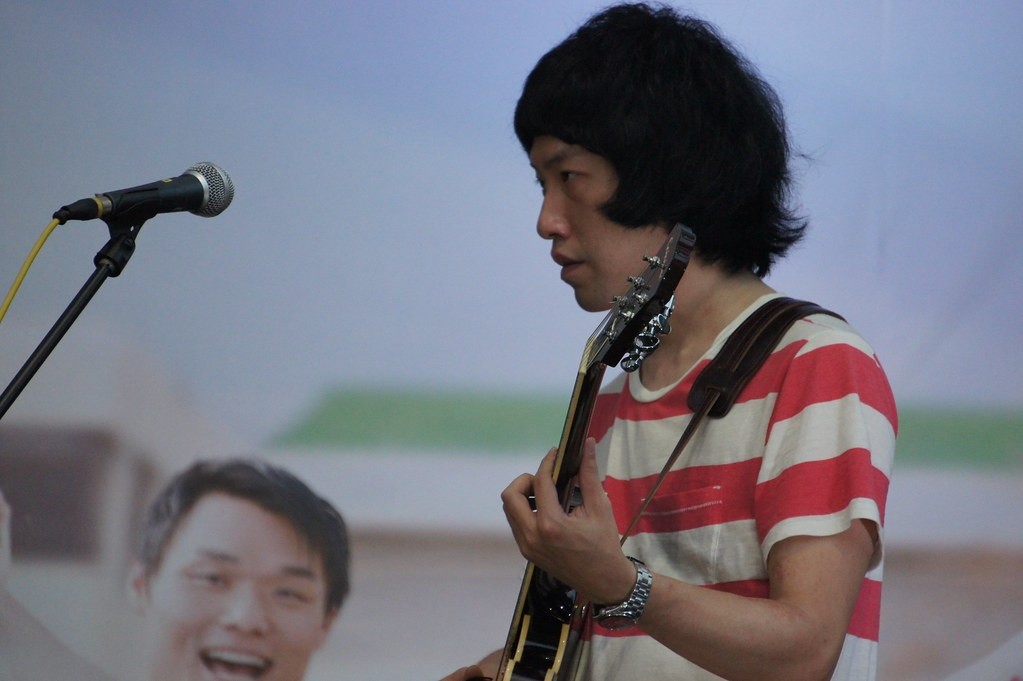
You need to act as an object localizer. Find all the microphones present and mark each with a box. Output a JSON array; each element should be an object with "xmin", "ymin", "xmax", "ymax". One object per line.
[{"xmin": 49, "ymin": 161, "xmax": 235, "ymax": 225}]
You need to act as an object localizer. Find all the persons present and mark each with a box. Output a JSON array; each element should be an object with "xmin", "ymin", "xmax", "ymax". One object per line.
[
  {"xmin": 127, "ymin": 451, "xmax": 348, "ymax": 681},
  {"xmin": 438, "ymin": 2, "xmax": 898, "ymax": 680}
]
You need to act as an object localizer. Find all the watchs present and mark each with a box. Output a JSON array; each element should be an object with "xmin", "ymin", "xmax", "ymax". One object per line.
[{"xmin": 591, "ymin": 556, "xmax": 653, "ymax": 631}]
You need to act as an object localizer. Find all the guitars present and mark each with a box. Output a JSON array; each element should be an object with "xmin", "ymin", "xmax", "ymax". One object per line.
[{"xmin": 493, "ymin": 224, "xmax": 695, "ymax": 681}]
